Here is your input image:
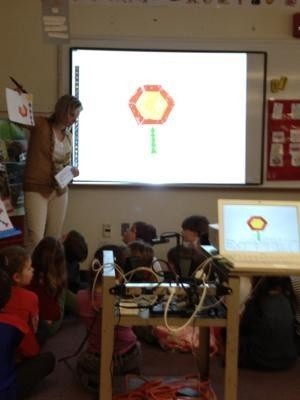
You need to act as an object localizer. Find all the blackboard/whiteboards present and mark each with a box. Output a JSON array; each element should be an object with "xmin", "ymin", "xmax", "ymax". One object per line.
[{"xmin": 59, "ymin": 37, "xmax": 300, "ymax": 189}]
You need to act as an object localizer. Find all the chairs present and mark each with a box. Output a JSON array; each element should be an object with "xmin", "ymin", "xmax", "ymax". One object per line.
[{"xmin": 0, "ymin": 314, "xmax": 30, "ymax": 400}]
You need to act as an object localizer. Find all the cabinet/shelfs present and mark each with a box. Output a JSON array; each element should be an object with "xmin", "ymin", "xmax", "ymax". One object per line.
[
  {"xmin": 97, "ymin": 248, "xmax": 240, "ymax": 400},
  {"xmin": 0, "ymin": 138, "xmax": 31, "ymax": 252}
]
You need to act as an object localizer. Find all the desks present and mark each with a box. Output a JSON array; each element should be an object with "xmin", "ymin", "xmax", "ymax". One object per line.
[{"xmin": 203, "ymin": 243, "xmax": 299, "ymax": 399}]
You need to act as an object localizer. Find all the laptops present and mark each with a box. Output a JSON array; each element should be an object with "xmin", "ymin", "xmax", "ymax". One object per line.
[{"xmin": 218, "ymin": 198, "xmax": 300, "ymax": 270}]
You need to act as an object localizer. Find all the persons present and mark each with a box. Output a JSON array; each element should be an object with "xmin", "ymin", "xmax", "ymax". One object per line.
[{"xmin": 14, "ymin": 85, "xmax": 82, "ymax": 250}]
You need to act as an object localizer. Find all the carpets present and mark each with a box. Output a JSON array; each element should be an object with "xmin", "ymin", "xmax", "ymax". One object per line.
[{"xmin": 124, "ymin": 373, "xmax": 218, "ymax": 400}]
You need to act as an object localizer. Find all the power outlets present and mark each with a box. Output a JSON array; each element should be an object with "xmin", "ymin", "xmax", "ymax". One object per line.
[{"xmin": 101, "ymin": 224, "xmax": 111, "ymax": 237}]
